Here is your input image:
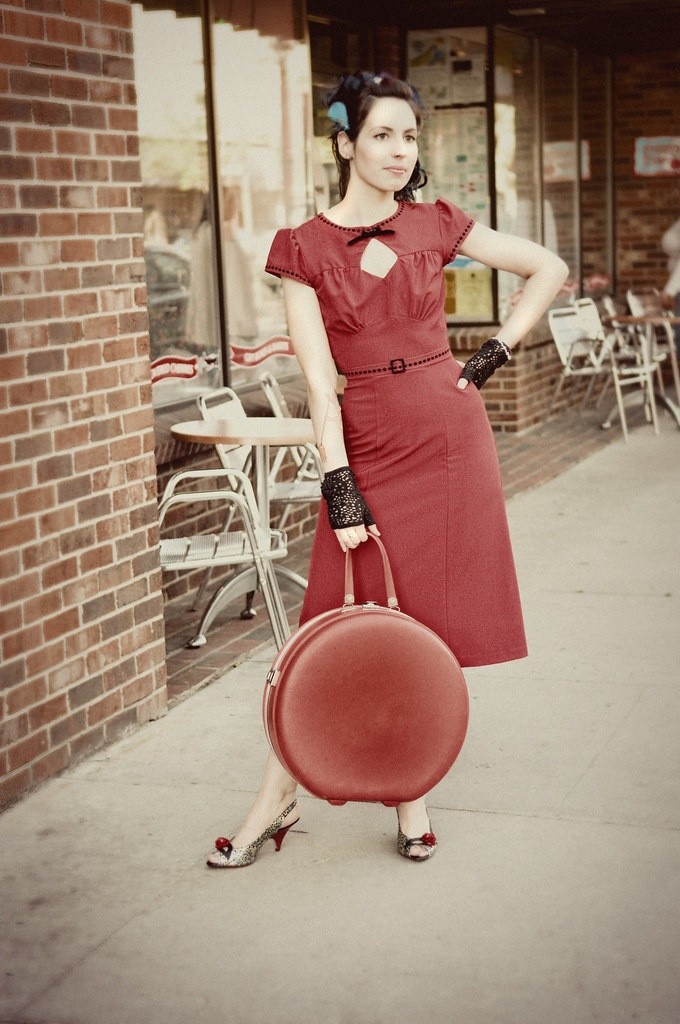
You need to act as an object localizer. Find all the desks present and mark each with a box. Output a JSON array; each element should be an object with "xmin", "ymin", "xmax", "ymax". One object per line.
[
  {"xmin": 168, "ymin": 415, "xmax": 319, "ymax": 645},
  {"xmin": 602, "ymin": 312, "xmax": 680, "ymax": 430}
]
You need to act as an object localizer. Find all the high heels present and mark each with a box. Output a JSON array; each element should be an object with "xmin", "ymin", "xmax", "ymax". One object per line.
[
  {"xmin": 207, "ymin": 799, "xmax": 299, "ymax": 869},
  {"xmin": 395, "ymin": 805, "xmax": 438, "ymax": 861}
]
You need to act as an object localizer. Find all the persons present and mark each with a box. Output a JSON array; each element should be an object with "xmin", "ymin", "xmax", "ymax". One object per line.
[
  {"xmin": 660, "ymin": 217, "xmax": 680, "ymax": 375},
  {"xmin": 205, "ymin": 72, "xmax": 571, "ymax": 869},
  {"xmin": 187, "ymin": 186, "xmax": 257, "ymax": 354}
]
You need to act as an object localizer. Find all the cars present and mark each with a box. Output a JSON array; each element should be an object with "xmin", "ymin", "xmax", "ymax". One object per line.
[{"xmin": 141, "ymin": 244, "xmax": 191, "ymax": 356}]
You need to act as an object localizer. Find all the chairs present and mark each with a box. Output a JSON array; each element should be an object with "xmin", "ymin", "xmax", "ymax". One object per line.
[
  {"xmin": 151, "ymin": 371, "xmax": 327, "ymax": 650},
  {"xmin": 546, "ymin": 283, "xmax": 680, "ymax": 443}
]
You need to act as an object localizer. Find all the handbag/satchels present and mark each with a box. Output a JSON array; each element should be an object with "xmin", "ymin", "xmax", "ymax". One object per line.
[{"xmin": 262, "ymin": 532, "xmax": 468, "ymax": 805}]
